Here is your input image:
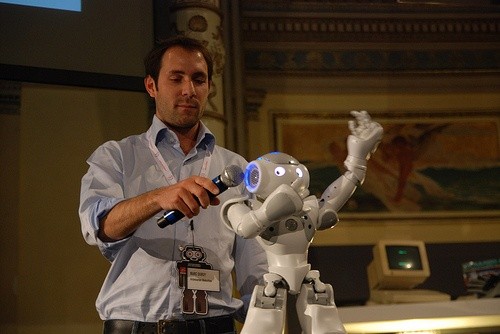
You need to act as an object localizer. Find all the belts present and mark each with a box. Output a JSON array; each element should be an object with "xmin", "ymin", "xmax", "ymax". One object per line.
[{"xmin": 103, "ymin": 315, "xmax": 235, "ymax": 334}]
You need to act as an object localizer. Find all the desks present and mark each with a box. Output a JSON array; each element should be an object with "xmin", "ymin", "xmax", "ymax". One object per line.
[{"xmin": 337, "ymin": 298, "xmax": 500, "ymax": 334}]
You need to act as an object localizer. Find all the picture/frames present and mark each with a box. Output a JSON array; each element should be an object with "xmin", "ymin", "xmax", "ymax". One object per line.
[{"xmin": 273, "ymin": 112, "xmax": 500, "ymax": 220}]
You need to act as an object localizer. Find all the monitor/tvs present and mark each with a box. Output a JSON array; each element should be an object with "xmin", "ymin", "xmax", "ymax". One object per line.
[{"xmin": 367, "ymin": 239, "xmax": 431, "ymax": 290}]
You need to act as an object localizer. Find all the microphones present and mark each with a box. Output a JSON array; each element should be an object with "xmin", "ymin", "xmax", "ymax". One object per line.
[{"xmin": 156, "ymin": 164, "xmax": 245, "ymax": 229}]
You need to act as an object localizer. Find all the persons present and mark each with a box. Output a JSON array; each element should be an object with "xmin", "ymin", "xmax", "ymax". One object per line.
[
  {"xmin": 219, "ymin": 108, "xmax": 383, "ymax": 333},
  {"xmin": 78, "ymin": 37, "xmax": 269, "ymax": 334}
]
